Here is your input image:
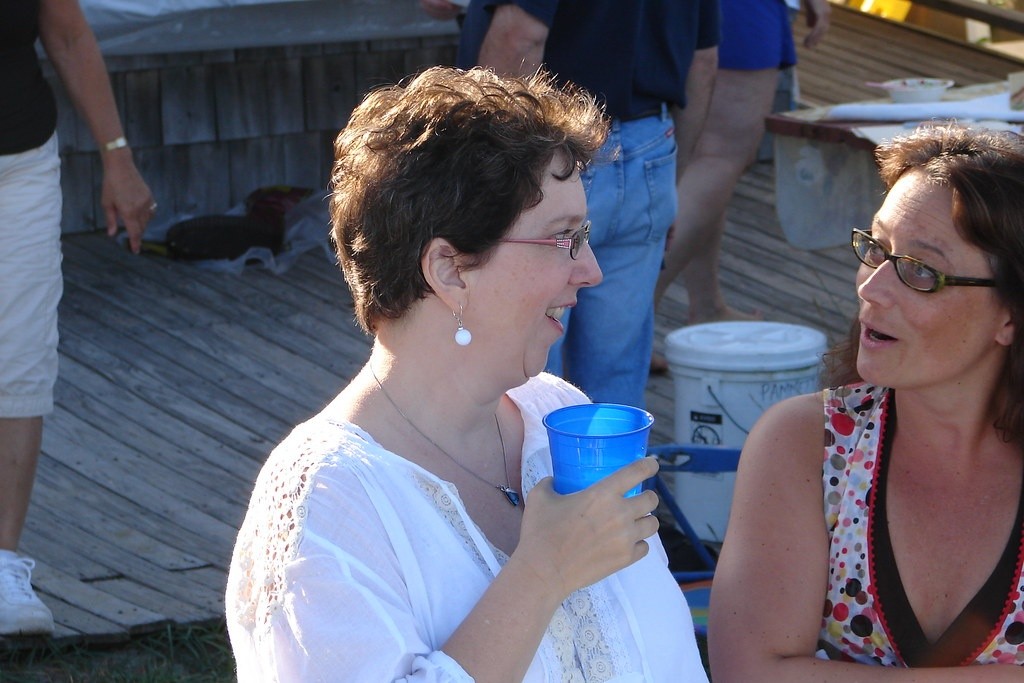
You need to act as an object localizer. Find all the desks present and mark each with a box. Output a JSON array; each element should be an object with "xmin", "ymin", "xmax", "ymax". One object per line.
[{"xmin": 764, "ymin": 92, "xmax": 1023, "ymax": 251}]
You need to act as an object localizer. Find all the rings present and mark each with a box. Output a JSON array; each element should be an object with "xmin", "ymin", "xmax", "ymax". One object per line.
[{"xmin": 149, "ymin": 202, "xmax": 157, "ymax": 210}]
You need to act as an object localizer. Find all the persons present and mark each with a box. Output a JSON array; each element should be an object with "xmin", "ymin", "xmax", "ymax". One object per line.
[
  {"xmin": 225, "ymin": 67, "xmax": 708, "ymax": 683},
  {"xmin": 456, "ymin": 0, "xmax": 833, "ymax": 408},
  {"xmin": 709, "ymin": 121, "xmax": 1024, "ymax": 683},
  {"xmin": 1, "ymin": 1, "xmax": 157, "ymax": 639}
]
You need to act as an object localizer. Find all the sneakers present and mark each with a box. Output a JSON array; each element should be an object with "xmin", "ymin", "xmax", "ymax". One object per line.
[{"xmin": 0, "ymin": 556, "xmax": 54, "ymax": 635}]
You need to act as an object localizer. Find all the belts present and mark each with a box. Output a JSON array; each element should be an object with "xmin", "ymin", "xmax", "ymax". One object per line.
[{"xmin": 607, "ymin": 105, "xmax": 662, "ymax": 125}]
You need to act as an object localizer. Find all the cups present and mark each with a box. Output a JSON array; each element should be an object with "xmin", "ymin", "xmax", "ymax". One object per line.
[{"xmin": 542, "ymin": 403, "xmax": 655, "ymax": 499}]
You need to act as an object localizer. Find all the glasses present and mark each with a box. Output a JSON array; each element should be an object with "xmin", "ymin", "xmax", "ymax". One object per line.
[
  {"xmin": 496, "ymin": 221, "xmax": 591, "ymax": 260},
  {"xmin": 850, "ymin": 227, "xmax": 999, "ymax": 294}
]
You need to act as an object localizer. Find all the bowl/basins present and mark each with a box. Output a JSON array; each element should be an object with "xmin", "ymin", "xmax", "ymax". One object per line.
[{"xmin": 882, "ymin": 77, "xmax": 954, "ymax": 103}]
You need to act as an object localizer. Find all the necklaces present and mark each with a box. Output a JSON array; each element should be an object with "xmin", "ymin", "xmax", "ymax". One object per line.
[{"xmin": 368, "ymin": 362, "xmax": 524, "ymax": 511}]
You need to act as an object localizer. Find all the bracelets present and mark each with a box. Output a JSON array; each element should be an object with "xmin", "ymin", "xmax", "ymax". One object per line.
[{"xmin": 97, "ymin": 136, "xmax": 128, "ymax": 154}]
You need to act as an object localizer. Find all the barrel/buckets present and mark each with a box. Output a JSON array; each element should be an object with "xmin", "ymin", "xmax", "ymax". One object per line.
[{"xmin": 664, "ymin": 321, "xmax": 828, "ymax": 541}]
